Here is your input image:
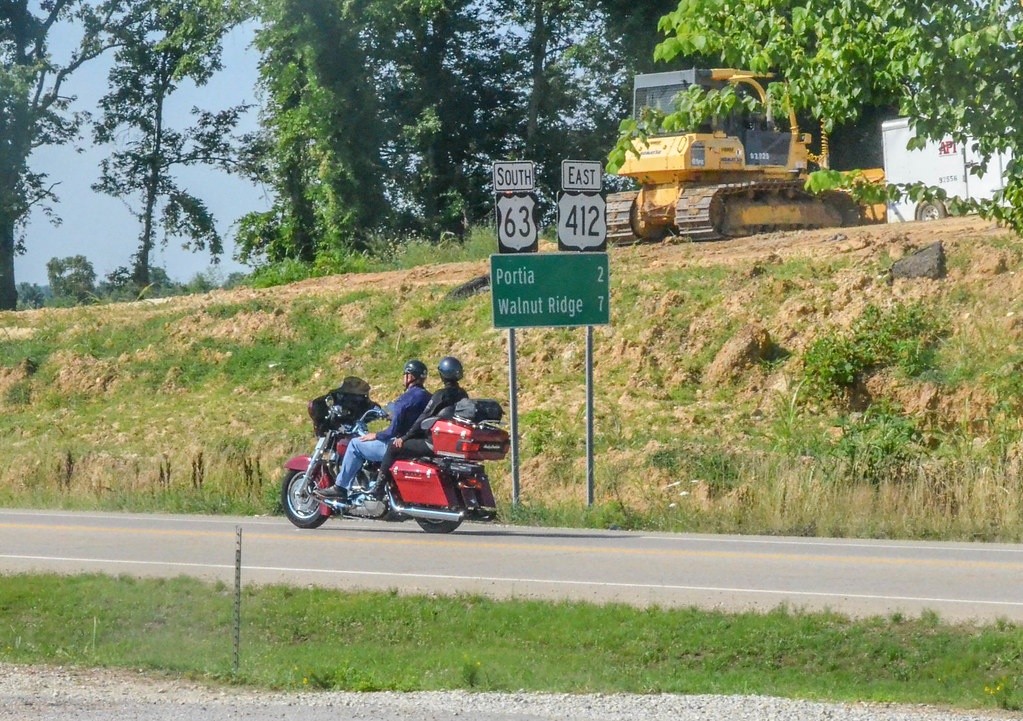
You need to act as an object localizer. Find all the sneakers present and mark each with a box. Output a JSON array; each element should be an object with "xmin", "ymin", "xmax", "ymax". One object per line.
[{"xmin": 313, "ymin": 485, "xmax": 347, "ymax": 501}]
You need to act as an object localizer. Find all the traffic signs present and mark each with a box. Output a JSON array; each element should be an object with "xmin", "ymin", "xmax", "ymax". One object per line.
[{"xmin": 490, "ymin": 252, "xmax": 611, "ymax": 327}]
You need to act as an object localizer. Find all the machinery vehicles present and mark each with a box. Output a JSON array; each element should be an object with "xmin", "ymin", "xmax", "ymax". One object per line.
[{"xmin": 605, "ymin": 65, "xmax": 874, "ymax": 244}]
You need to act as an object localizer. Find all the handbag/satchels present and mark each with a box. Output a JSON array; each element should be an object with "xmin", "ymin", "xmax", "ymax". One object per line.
[{"xmin": 456, "ymin": 396, "xmax": 505, "ymax": 424}]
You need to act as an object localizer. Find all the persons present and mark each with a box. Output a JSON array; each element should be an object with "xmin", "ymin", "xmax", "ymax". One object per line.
[
  {"xmin": 313, "ymin": 360, "xmax": 433, "ymax": 499},
  {"xmin": 359, "ymin": 357, "xmax": 469, "ymax": 494}
]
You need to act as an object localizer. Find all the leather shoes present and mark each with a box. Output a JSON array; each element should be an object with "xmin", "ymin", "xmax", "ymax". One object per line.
[{"xmin": 360, "ymin": 484, "xmax": 386, "ymax": 502}]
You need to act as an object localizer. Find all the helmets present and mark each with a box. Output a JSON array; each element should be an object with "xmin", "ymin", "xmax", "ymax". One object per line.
[
  {"xmin": 437, "ymin": 356, "xmax": 464, "ymax": 382},
  {"xmin": 403, "ymin": 361, "xmax": 428, "ymax": 379}
]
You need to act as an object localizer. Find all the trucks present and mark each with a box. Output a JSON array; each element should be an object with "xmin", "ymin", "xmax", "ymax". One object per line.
[{"xmin": 880, "ymin": 107, "xmax": 1016, "ymax": 223}]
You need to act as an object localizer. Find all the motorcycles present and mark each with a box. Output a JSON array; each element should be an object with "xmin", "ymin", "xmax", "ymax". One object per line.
[{"xmin": 278, "ymin": 377, "xmax": 509, "ymax": 534}]
